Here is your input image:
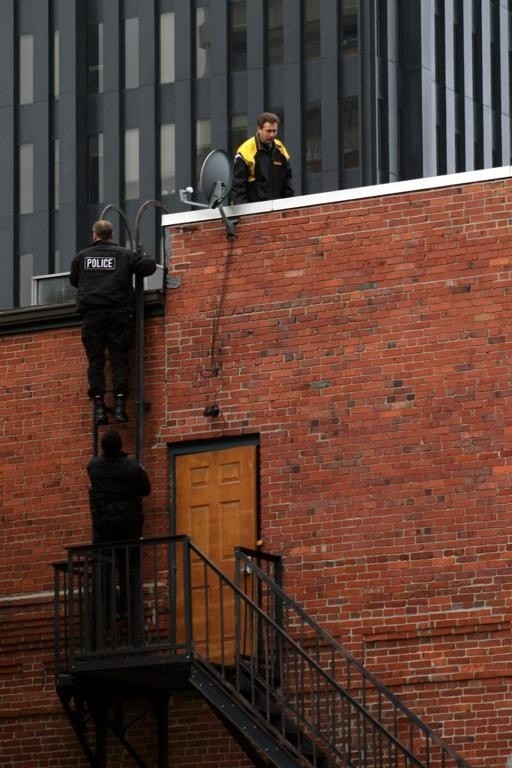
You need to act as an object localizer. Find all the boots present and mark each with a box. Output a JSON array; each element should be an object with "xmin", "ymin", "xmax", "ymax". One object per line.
[
  {"xmin": 114, "ymin": 394, "xmax": 130, "ymax": 422},
  {"xmin": 94, "ymin": 395, "xmax": 109, "ymax": 425}
]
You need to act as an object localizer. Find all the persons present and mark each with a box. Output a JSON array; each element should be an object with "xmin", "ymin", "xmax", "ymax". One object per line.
[
  {"xmin": 70, "ymin": 220, "xmax": 156, "ymax": 423},
  {"xmin": 84, "ymin": 429, "xmax": 154, "ymax": 652},
  {"xmin": 230, "ymin": 112, "xmax": 296, "ymax": 203}
]
